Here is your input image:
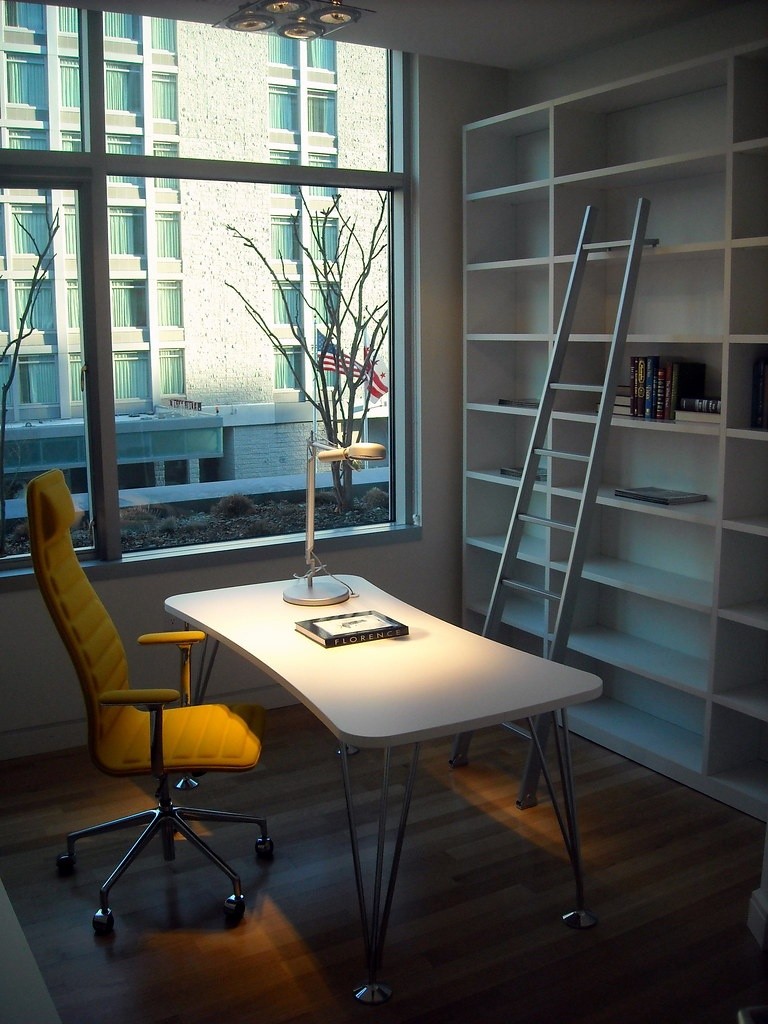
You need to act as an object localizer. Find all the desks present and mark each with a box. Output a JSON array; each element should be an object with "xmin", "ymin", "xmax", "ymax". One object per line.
[{"xmin": 164, "ymin": 574, "xmax": 604, "ymax": 1003}]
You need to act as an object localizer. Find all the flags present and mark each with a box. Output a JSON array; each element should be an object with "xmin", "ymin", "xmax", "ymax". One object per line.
[
  {"xmin": 315, "ymin": 329, "xmax": 371, "ymax": 381},
  {"xmin": 365, "ymin": 324, "xmax": 388, "ymax": 403}
]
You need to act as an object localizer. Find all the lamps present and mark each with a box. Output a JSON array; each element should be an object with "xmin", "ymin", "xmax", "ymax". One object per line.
[
  {"xmin": 212, "ymin": 0, "xmax": 375, "ymax": 43},
  {"xmin": 283, "ymin": 430, "xmax": 386, "ymax": 606}
]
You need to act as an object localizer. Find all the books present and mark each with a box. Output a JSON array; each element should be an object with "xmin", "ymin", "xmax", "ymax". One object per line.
[
  {"xmin": 294, "ymin": 611, "xmax": 409, "ymax": 648},
  {"xmin": 497, "ymin": 353, "xmax": 768, "ymax": 506}
]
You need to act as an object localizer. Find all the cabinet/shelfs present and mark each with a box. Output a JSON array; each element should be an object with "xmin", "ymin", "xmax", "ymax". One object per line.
[{"xmin": 462, "ymin": 37, "xmax": 768, "ymax": 823}]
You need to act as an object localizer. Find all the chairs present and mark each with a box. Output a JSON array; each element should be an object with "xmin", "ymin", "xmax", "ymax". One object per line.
[{"xmin": 26, "ymin": 468, "xmax": 274, "ymax": 933}]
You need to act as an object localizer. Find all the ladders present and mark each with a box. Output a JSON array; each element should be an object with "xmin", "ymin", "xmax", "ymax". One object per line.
[{"xmin": 447, "ymin": 194, "xmax": 660, "ymax": 807}]
